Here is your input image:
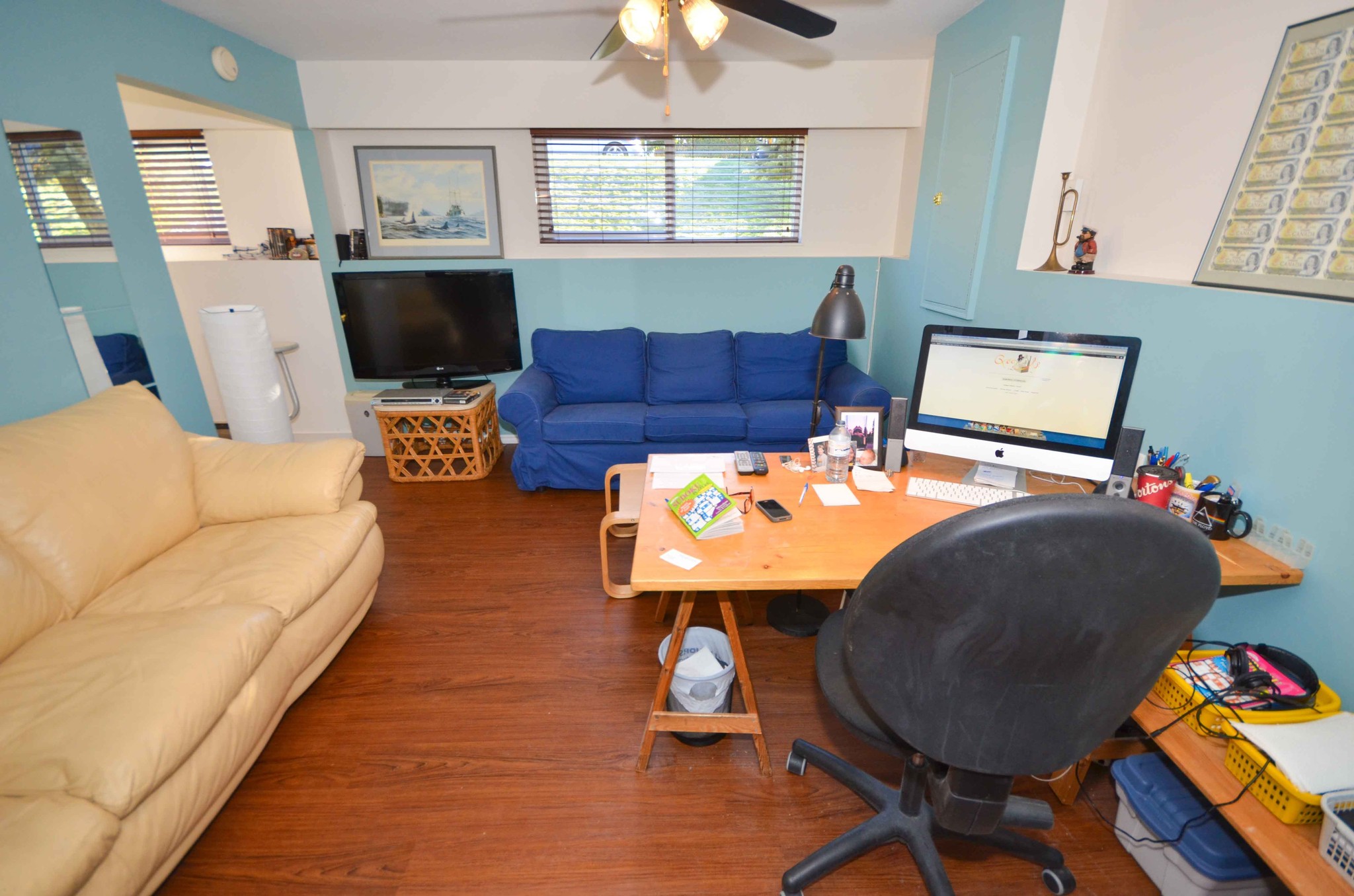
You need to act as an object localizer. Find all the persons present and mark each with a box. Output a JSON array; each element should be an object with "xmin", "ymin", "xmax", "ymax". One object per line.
[
  {"xmin": 855, "ymin": 449, "xmax": 877, "ymax": 466},
  {"xmin": 851, "ymin": 425, "xmax": 867, "ymax": 449},
  {"xmin": 848, "ymin": 446, "xmax": 855, "ymax": 465},
  {"xmin": 816, "ymin": 445, "xmax": 828, "ymax": 467}
]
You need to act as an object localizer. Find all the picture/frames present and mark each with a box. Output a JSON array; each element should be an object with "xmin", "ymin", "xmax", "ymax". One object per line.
[{"xmin": 352, "ymin": 144, "xmax": 505, "ymax": 262}]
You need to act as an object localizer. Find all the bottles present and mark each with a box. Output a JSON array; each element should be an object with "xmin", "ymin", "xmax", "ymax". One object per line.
[{"xmin": 826, "ymin": 420, "xmax": 851, "ymax": 483}]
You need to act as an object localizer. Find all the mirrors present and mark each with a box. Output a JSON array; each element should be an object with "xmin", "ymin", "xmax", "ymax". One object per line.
[{"xmin": 1, "ymin": 119, "xmax": 162, "ymax": 400}]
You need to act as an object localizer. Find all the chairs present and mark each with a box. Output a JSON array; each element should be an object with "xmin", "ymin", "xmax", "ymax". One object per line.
[{"xmin": 771, "ymin": 491, "xmax": 1226, "ymax": 896}]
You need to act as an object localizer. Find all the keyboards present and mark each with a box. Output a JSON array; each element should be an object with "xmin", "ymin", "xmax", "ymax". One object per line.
[{"xmin": 906, "ymin": 477, "xmax": 1037, "ymax": 507}]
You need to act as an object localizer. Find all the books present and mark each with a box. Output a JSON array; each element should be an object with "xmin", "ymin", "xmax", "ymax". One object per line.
[
  {"xmin": 667, "ymin": 474, "xmax": 744, "ymax": 540},
  {"xmin": 1171, "ymin": 647, "xmax": 1306, "ymax": 709}
]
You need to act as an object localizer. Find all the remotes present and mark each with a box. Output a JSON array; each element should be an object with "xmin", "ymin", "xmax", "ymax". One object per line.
[
  {"xmin": 749, "ymin": 452, "xmax": 769, "ymax": 476},
  {"xmin": 734, "ymin": 450, "xmax": 754, "ymax": 476}
]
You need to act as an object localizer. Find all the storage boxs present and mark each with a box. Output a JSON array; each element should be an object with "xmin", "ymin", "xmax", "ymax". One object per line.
[{"xmin": 1109, "ymin": 752, "xmax": 1293, "ymax": 896}]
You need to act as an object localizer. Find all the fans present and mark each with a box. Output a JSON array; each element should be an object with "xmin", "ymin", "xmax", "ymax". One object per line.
[{"xmin": 590, "ymin": 2, "xmax": 840, "ymax": 61}]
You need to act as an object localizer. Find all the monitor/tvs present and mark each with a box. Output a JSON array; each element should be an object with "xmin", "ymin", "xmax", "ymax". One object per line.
[
  {"xmin": 903, "ymin": 325, "xmax": 1141, "ymax": 482},
  {"xmin": 331, "ymin": 269, "xmax": 524, "ymax": 380}
]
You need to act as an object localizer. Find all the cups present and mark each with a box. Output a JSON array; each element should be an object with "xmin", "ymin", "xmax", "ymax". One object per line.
[{"xmin": 1136, "ymin": 465, "xmax": 1253, "ymax": 541}]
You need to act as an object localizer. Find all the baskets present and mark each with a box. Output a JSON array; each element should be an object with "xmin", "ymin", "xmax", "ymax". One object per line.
[
  {"xmin": 1154, "ymin": 644, "xmax": 1340, "ymax": 736},
  {"xmin": 1217, "ymin": 711, "xmax": 1354, "ymax": 824},
  {"xmin": 1317, "ymin": 789, "xmax": 1354, "ymax": 890}
]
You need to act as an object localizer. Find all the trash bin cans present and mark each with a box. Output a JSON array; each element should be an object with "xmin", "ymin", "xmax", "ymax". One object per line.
[{"xmin": 659, "ymin": 626, "xmax": 737, "ymax": 747}]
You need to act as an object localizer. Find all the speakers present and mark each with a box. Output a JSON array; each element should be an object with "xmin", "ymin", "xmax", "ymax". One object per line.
[
  {"xmin": 1105, "ymin": 427, "xmax": 1145, "ymax": 499},
  {"xmin": 884, "ymin": 397, "xmax": 908, "ymax": 473}
]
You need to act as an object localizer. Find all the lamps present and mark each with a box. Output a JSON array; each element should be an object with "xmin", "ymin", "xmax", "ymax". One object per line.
[
  {"xmin": 618, "ymin": 1, "xmax": 731, "ymax": 117},
  {"xmin": 801, "ymin": 265, "xmax": 865, "ymax": 448}
]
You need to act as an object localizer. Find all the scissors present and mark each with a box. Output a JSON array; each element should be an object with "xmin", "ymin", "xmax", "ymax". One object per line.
[{"xmin": 1174, "ymin": 466, "xmax": 1186, "ymax": 488}]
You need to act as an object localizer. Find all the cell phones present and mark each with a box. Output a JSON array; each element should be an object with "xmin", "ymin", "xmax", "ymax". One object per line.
[
  {"xmin": 756, "ymin": 498, "xmax": 792, "ymax": 523},
  {"xmin": 779, "ymin": 455, "xmax": 792, "ymax": 465}
]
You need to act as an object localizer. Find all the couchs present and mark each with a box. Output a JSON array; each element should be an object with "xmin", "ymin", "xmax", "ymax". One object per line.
[
  {"xmin": 0, "ymin": 378, "xmax": 389, "ymax": 896},
  {"xmin": 498, "ymin": 325, "xmax": 894, "ymax": 492}
]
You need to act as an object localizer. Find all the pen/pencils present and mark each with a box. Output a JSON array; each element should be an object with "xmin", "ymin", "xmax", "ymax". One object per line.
[
  {"xmin": 1147, "ymin": 445, "xmax": 1190, "ymax": 470},
  {"xmin": 798, "ymin": 482, "xmax": 808, "ymax": 507}
]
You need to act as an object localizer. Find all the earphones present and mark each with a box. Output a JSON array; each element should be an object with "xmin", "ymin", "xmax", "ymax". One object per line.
[{"xmin": 795, "ymin": 466, "xmax": 811, "ymax": 473}]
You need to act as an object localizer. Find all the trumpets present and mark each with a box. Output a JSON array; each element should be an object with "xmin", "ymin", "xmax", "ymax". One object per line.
[{"xmin": 1030, "ymin": 170, "xmax": 1079, "ymax": 274}]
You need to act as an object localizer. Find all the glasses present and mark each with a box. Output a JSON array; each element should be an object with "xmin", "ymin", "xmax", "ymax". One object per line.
[{"xmin": 725, "ymin": 486, "xmax": 754, "ymax": 514}]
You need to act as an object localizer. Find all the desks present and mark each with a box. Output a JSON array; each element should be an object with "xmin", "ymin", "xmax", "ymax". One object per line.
[
  {"xmin": 1130, "ymin": 649, "xmax": 1354, "ymax": 895},
  {"xmin": 625, "ymin": 451, "xmax": 1305, "ymax": 783}
]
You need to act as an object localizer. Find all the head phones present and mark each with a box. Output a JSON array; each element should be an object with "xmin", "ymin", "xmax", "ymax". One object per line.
[{"xmin": 1224, "ymin": 642, "xmax": 1321, "ymax": 708}]
